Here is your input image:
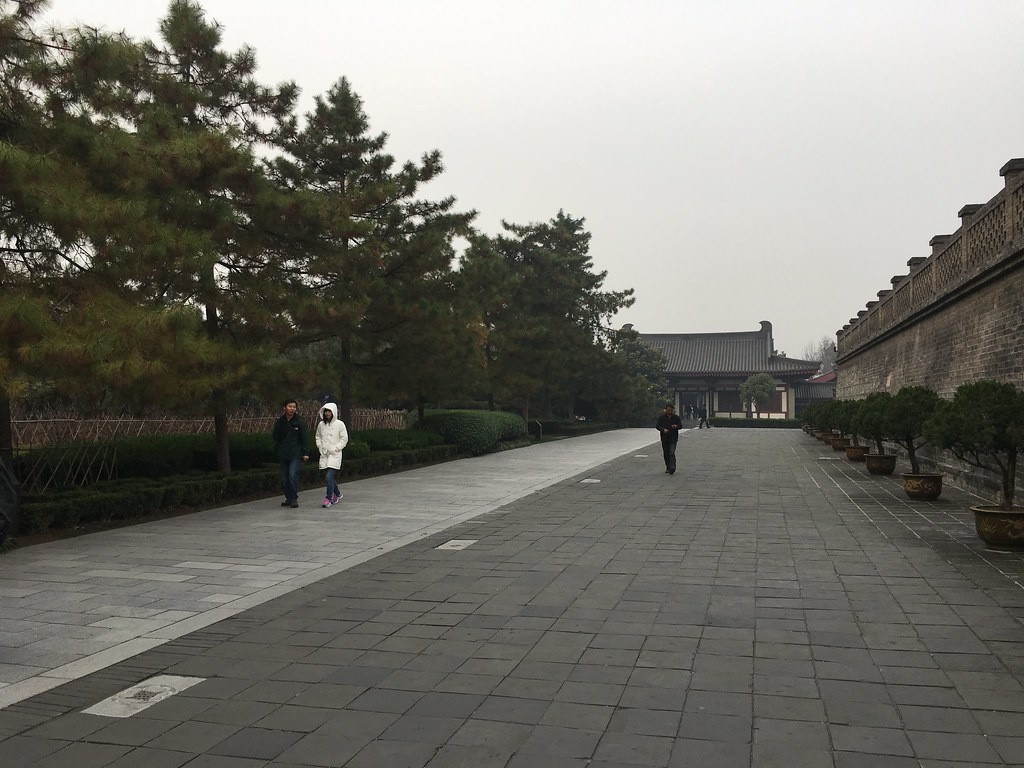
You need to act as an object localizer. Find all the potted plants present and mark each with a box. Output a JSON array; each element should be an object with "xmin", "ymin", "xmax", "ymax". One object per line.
[
  {"xmin": 922, "ymin": 379, "xmax": 1023, "ymax": 551},
  {"xmin": 798, "ymin": 385, "xmax": 945, "ymax": 502}
]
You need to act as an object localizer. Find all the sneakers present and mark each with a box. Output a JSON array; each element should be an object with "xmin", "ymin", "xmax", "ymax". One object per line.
[
  {"xmin": 331, "ymin": 494, "xmax": 343, "ymax": 505},
  {"xmin": 321, "ymin": 499, "xmax": 332, "ymax": 509}
]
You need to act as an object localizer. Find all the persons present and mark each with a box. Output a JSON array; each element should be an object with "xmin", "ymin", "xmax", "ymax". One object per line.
[
  {"xmin": 685, "ymin": 402, "xmax": 698, "ymax": 419},
  {"xmin": 656, "ymin": 404, "xmax": 682, "ymax": 475},
  {"xmin": 699, "ymin": 404, "xmax": 711, "ymax": 429},
  {"xmin": 315, "ymin": 403, "xmax": 348, "ymax": 508},
  {"xmin": 272, "ymin": 399, "xmax": 311, "ymax": 508}
]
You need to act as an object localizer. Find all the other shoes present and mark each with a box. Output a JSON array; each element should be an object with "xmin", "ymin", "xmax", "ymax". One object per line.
[
  {"xmin": 281, "ymin": 499, "xmax": 291, "ymax": 506},
  {"xmin": 665, "ymin": 469, "xmax": 674, "ymax": 476},
  {"xmin": 707, "ymin": 427, "xmax": 710, "ymax": 428},
  {"xmin": 699, "ymin": 427, "xmax": 703, "ymax": 429},
  {"xmin": 291, "ymin": 501, "xmax": 299, "ymax": 508}
]
